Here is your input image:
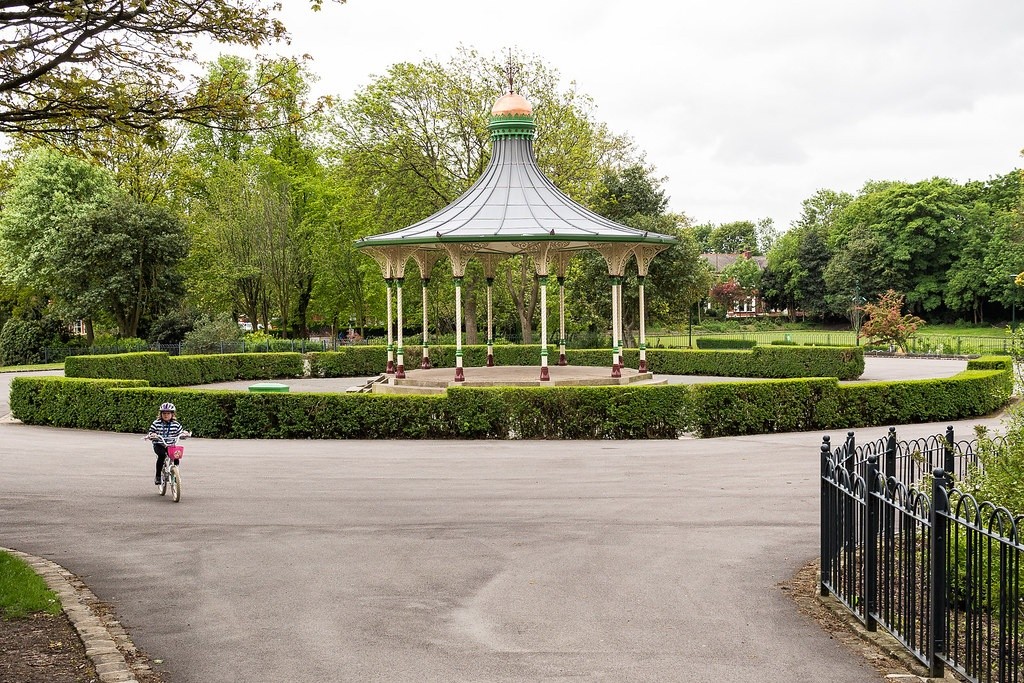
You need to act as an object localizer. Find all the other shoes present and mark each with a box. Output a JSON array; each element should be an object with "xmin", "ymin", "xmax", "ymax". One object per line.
[{"xmin": 155, "ymin": 481, "xmax": 161, "ymax": 485}]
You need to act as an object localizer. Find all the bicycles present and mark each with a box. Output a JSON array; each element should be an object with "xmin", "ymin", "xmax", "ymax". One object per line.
[{"xmin": 144, "ymin": 430, "xmax": 193, "ymax": 502}]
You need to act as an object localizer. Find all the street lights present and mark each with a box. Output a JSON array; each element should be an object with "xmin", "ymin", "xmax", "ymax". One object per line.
[{"xmin": 1007, "ymin": 274, "xmax": 1021, "ymax": 345}]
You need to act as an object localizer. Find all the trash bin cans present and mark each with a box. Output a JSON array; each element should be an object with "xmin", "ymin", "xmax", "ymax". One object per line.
[{"xmin": 248, "ymin": 383, "xmax": 290, "ymax": 392}]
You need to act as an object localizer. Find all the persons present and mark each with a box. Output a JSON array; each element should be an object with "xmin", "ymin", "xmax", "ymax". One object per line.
[{"xmin": 149, "ymin": 404, "xmax": 188, "ymax": 484}]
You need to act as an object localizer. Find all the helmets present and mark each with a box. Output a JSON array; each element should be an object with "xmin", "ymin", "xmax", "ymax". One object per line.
[{"xmin": 159, "ymin": 403, "xmax": 175, "ymax": 413}]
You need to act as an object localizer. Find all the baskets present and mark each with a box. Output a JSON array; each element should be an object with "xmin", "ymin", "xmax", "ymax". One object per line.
[{"xmin": 167, "ymin": 446, "xmax": 184, "ymax": 459}]
